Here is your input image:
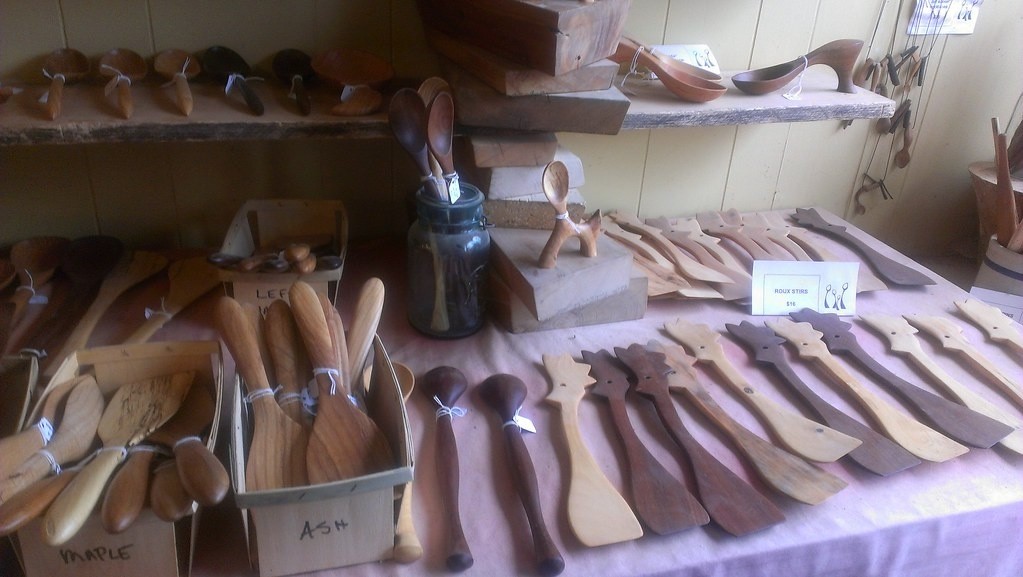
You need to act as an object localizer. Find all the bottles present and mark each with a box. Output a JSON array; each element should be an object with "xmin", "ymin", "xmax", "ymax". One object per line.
[{"xmin": 407, "ymin": 182, "xmax": 491, "ymax": 339}]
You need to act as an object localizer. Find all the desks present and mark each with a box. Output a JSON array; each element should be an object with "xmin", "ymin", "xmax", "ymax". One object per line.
[{"xmin": 0, "ymin": 210, "xmax": 1023, "ymax": 577}]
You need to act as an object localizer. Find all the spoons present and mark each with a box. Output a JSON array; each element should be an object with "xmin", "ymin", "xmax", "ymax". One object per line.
[
  {"xmin": 154, "ymin": 49, "xmax": 201, "ymax": 115},
  {"xmin": 42, "ymin": 48, "xmax": 91, "ymax": 120},
  {"xmin": 895, "ymin": 124, "xmax": 913, "ymax": 168},
  {"xmin": 421, "ymin": 366, "xmax": 473, "ymax": 572},
  {"xmin": 1, "ymin": 234, "xmax": 127, "ymax": 364},
  {"xmin": 272, "ymin": 48, "xmax": 319, "ymax": 116},
  {"xmin": 478, "ymin": 373, "xmax": 564, "ymax": 577},
  {"xmin": 877, "ymin": 85, "xmax": 892, "ymax": 135},
  {"xmin": 204, "ymin": 44, "xmax": 264, "ymax": 116},
  {"xmin": 99, "ymin": 48, "xmax": 147, "ymax": 120},
  {"xmin": 361, "ymin": 361, "xmax": 415, "ymax": 550},
  {"xmin": 390, "ymin": 76, "xmax": 480, "ymax": 332}
]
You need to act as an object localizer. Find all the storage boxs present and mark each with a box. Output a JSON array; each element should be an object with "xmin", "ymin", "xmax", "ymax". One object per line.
[
  {"xmin": 230, "ymin": 331, "xmax": 414, "ymax": 577},
  {"xmin": 418, "ymin": 0, "xmax": 626, "ymax": 76},
  {"xmin": 19, "ymin": 339, "xmax": 225, "ymax": 577},
  {"xmin": 213, "ymin": 197, "xmax": 352, "ymax": 316}
]
